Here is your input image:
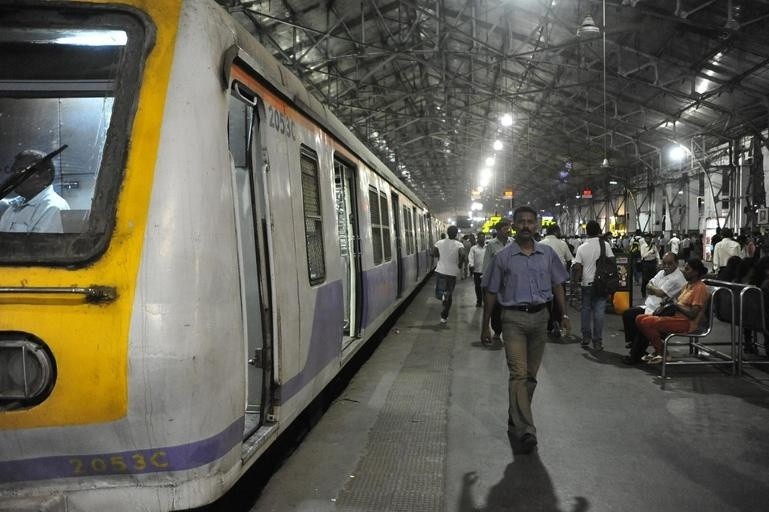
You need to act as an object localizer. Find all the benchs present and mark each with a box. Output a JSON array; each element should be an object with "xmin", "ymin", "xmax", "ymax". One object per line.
[
  {"xmin": 657, "ymin": 285, "xmax": 737, "ymax": 380},
  {"xmin": 699, "ymin": 278, "xmax": 769, "ymax": 378}
]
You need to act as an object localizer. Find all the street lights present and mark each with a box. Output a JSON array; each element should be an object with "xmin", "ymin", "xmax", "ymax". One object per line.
[
  {"xmin": 611, "ymin": 177, "xmax": 642, "ymax": 235},
  {"xmin": 552, "ymin": 201, "xmax": 574, "ymax": 240},
  {"xmin": 668, "ymin": 144, "xmax": 722, "ymax": 228}
]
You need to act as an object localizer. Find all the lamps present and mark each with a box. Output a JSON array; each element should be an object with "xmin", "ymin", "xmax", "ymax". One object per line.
[{"xmin": 576, "ymin": 1, "xmax": 602, "ymax": 37}]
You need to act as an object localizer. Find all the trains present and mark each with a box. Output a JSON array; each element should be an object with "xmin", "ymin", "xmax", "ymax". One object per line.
[{"xmin": 0, "ymin": 0, "xmax": 452, "ymax": 511}]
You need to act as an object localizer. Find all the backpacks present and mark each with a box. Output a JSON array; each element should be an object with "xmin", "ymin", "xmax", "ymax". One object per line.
[
  {"xmin": 630, "ymin": 236, "xmax": 642, "ymax": 257},
  {"xmin": 594, "ymin": 239, "xmax": 619, "ymax": 296}
]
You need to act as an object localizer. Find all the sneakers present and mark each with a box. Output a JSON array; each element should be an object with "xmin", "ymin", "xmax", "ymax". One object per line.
[
  {"xmin": 547, "ymin": 320, "xmax": 562, "ymax": 338},
  {"xmin": 623, "ymin": 342, "xmax": 673, "ymax": 365},
  {"xmin": 592, "ymin": 339, "xmax": 604, "ymax": 352},
  {"xmin": 439, "ymin": 317, "xmax": 449, "ymax": 324},
  {"xmin": 580, "ymin": 336, "xmax": 593, "ymax": 346},
  {"xmin": 506, "ymin": 429, "xmax": 538, "ymax": 455}
]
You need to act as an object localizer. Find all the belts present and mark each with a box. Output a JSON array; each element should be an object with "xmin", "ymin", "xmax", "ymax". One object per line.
[{"xmin": 503, "ymin": 304, "xmax": 546, "ymax": 312}]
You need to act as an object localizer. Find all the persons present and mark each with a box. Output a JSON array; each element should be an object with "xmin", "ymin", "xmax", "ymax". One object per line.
[
  {"xmin": 0, "ymin": 150, "xmax": 70, "ymax": 233},
  {"xmin": 481, "ymin": 207, "xmax": 571, "ymax": 456}
]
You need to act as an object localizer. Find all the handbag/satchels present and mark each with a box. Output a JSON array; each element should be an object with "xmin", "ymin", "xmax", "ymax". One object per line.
[{"xmin": 652, "ymin": 301, "xmax": 676, "ymax": 317}]
